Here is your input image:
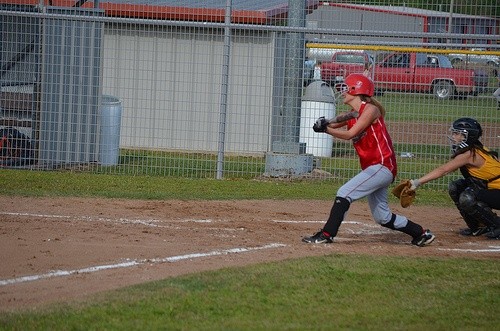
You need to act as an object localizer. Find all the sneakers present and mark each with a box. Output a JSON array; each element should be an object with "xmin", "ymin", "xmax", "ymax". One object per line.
[
  {"xmin": 482, "ymin": 228, "xmax": 500, "ymax": 240},
  {"xmin": 412, "ymin": 229, "xmax": 436, "ymax": 246},
  {"xmin": 459, "ymin": 226, "xmax": 492, "ymax": 236},
  {"xmin": 302, "ymin": 228, "xmax": 333, "ymax": 243}
]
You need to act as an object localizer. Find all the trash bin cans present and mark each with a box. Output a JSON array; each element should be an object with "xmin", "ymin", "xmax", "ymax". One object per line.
[
  {"xmin": 100, "ymin": 95, "xmax": 122, "ymax": 166},
  {"xmin": 299, "ymin": 80, "xmax": 336, "ymax": 158}
]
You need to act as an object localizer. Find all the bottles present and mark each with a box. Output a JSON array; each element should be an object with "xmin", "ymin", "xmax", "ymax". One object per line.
[{"xmin": 401, "ymin": 152, "xmax": 414, "ymax": 158}]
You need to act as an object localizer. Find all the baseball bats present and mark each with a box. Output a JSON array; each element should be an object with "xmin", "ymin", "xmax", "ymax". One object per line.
[{"xmin": 321, "ymin": 111, "xmax": 359, "ymax": 126}]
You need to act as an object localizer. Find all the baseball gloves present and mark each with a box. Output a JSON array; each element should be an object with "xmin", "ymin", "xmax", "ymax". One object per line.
[{"xmin": 392, "ymin": 180, "xmax": 415, "ymax": 209}]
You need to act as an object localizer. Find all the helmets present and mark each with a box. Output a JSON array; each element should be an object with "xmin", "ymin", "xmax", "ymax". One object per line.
[
  {"xmin": 344, "ymin": 74, "xmax": 374, "ymax": 96},
  {"xmin": 452, "ymin": 117, "xmax": 483, "ymax": 148}
]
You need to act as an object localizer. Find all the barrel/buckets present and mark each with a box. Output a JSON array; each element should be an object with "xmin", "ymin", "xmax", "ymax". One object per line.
[{"xmin": 98, "ymin": 94, "xmax": 122, "ymax": 166}]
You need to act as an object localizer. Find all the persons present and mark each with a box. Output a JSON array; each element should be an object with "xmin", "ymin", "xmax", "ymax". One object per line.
[
  {"xmin": 392, "ymin": 117, "xmax": 500, "ymax": 239},
  {"xmin": 301, "ymin": 73, "xmax": 435, "ymax": 246}
]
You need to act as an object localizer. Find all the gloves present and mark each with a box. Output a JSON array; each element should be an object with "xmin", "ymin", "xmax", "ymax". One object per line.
[{"xmin": 313, "ymin": 116, "xmax": 329, "ymax": 133}]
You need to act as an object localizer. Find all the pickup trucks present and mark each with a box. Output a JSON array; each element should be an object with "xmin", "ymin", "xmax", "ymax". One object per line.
[{"xmin": 304, "ymin": 49, "xmax": 500, "ymax": 99}]
